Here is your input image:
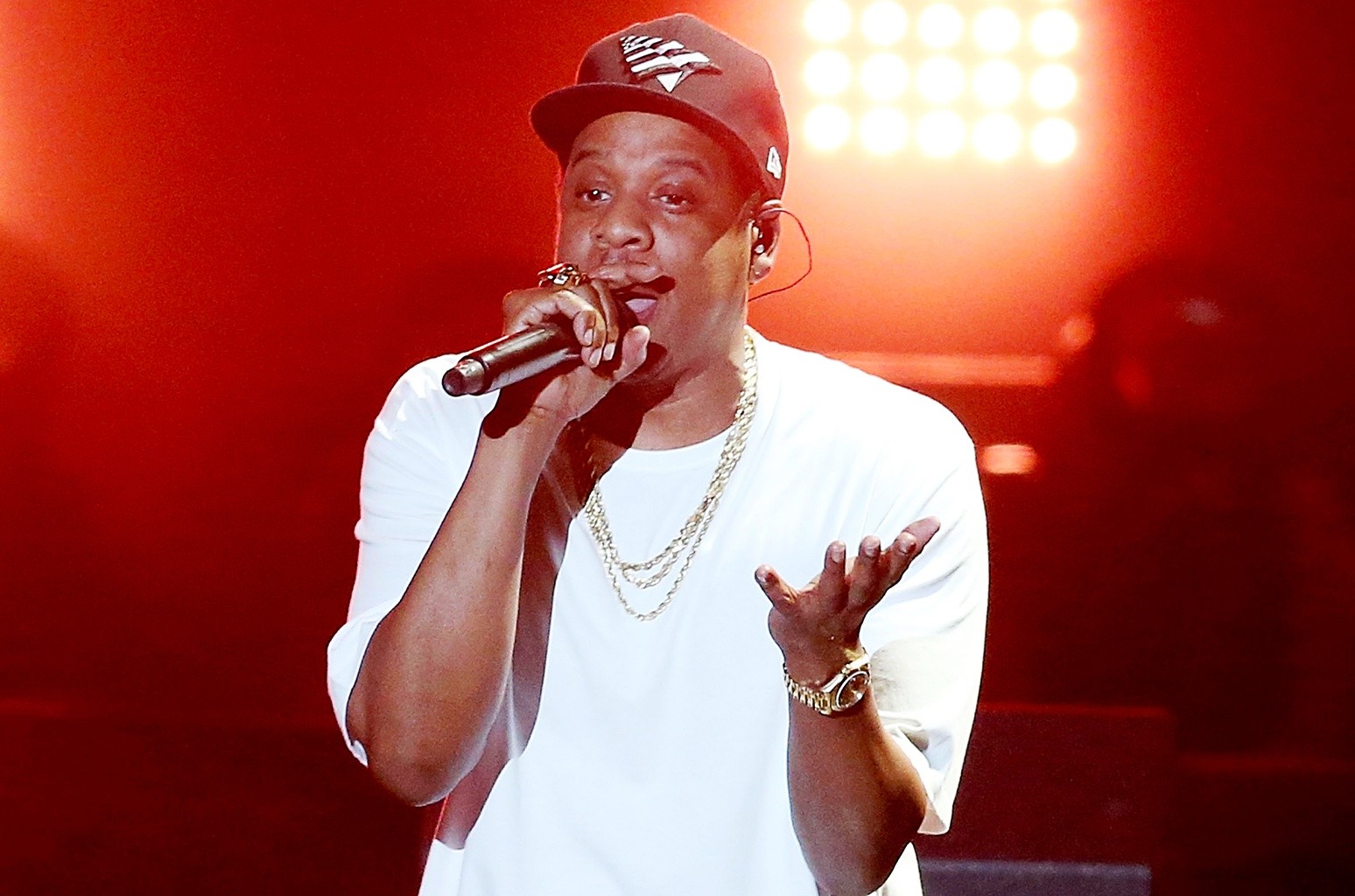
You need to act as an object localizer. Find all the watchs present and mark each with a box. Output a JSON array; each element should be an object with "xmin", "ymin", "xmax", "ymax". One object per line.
[{"xmin": 782, "ymin": 646, "xmax": 874, "ymax": 714}]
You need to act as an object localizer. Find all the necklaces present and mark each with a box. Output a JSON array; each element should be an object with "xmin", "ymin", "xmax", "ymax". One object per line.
[{"xmin": 556, "ymin": 328, "xmax": 759, "ymax": 622}]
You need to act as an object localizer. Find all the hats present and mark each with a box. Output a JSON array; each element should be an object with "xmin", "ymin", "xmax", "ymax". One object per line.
[{"xmin": 529, "ymin": 13, "xmax": 789, "ymax": 210}]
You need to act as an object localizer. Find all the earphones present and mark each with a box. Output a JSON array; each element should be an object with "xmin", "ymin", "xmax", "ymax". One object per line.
[{"xmin": 752, "ymin": 224, "xmax": 774, "ymax": 254}]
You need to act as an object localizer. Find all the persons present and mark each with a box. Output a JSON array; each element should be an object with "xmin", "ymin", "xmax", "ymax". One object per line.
[{"xmin": 328, "ymin": 14, "xmax": 989, "ymax": 896}]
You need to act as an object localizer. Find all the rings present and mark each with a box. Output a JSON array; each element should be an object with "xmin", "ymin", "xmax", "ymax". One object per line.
[{"xmin": 537, "ymin": 262, "xmax": 587, "ymax": 288}]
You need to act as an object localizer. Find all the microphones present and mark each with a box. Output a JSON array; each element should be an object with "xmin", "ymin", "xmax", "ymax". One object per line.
[{"xmin": 443, "ymin": 296, "xmax": 632, "ymax": 397}]
